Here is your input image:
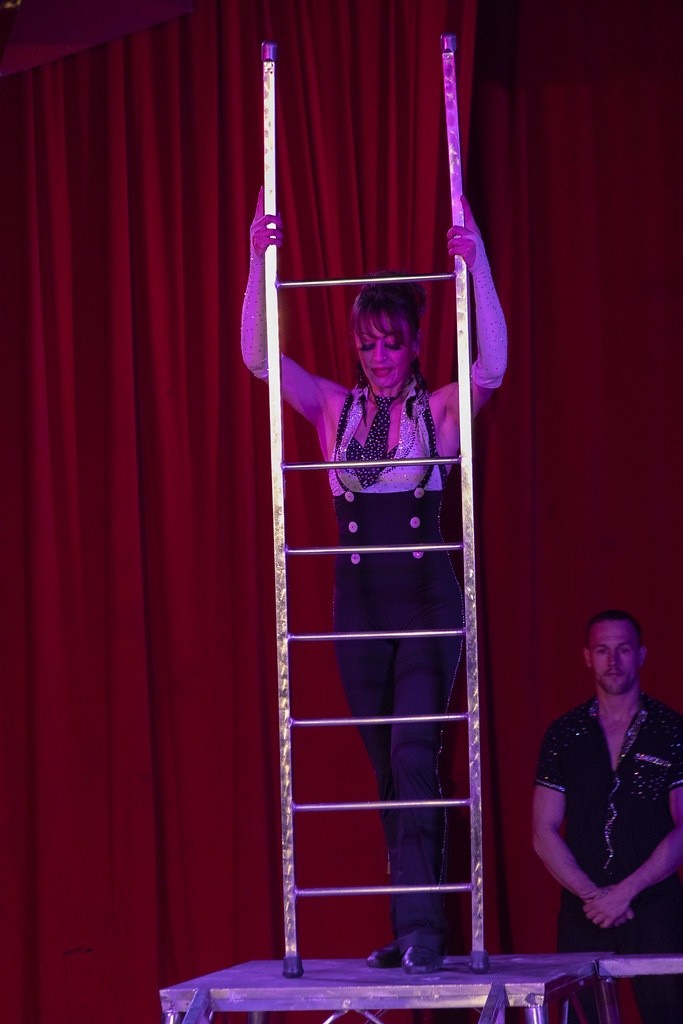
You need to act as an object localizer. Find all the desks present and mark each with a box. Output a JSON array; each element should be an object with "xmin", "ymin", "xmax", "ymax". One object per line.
[{"xmin": 157, "ymin": 950, "xmax": 622, "ymax": 1024}]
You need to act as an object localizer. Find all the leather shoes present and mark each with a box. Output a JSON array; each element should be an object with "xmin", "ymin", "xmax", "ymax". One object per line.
[
  {"xmin": 366, "ymin": 945, "xmax": 402, "ymax": 968},
  {"xmin": 401, "ymin": 946, "xmax": 444, "ymax": 973}
]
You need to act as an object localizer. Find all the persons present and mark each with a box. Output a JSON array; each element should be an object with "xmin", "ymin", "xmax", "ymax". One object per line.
[
  {"xmin": 242, "ymin": 184, "xmax": 506, "ymax": 975},
  {"xmin": 531, "ymin": 609, "xmax": 683, "ymax": 1024}
]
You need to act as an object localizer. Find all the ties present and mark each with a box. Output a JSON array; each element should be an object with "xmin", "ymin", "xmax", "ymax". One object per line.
[{"xmin": 355, "ymin": 395, "xmax": 396, "ymax": 489}]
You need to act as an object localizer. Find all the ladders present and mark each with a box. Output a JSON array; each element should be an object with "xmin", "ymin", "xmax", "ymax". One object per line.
[{"xmin": 260, "ymin": 30, "xmax": 490, "ymax": 980}]
